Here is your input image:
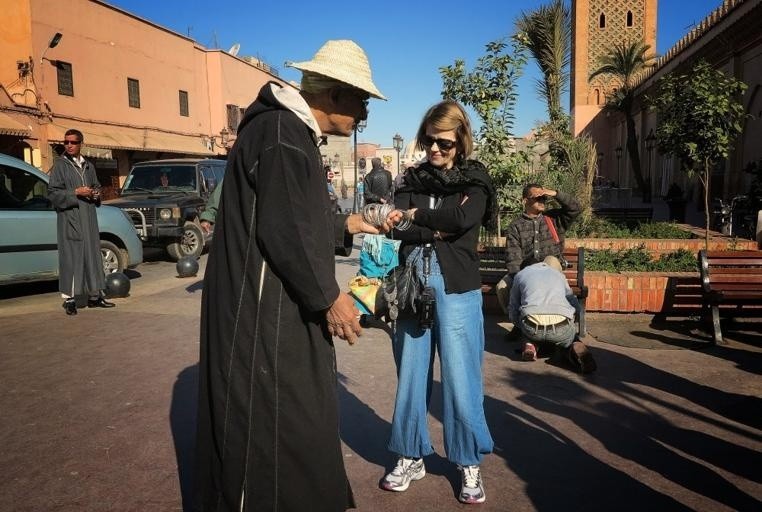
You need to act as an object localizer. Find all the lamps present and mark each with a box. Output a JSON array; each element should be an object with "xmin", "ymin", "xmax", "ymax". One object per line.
[{"xmin": 40, "ymin": 31, "xmax": 63, "ymax": 64}]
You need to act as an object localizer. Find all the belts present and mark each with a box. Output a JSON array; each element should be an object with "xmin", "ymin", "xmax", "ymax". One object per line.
[{"xmin": 522, "ymin": 318, "xmax": 573, "ymax": 333}]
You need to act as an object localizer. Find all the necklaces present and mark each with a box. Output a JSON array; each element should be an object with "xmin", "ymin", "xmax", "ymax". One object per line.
[{"xmin": 382, "ymin": 228, "xmax": 399, "ymax": 334}]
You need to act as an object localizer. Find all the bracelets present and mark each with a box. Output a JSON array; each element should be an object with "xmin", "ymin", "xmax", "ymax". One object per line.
[{"xmin": 362, "ymin": 204, "xmax": 412, "ymax": 231}]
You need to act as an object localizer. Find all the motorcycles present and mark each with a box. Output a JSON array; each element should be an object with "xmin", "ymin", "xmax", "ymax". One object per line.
[{"xmin": 713, "ymin": 194, "xmax": 757, "ymax": 238}]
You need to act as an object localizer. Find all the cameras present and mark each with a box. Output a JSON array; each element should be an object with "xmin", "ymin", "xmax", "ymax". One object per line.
[{"xmin": 413, "ymin": 287, "xmax": 437, "ymax": 329}]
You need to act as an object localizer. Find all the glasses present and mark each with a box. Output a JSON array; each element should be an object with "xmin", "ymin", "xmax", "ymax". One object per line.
[
  {"xmin": 341, "ymin": 91, "xmax": 372, "ymax": 110},
  {"xmin": 528, "ymin": 195, "xmax": 550, "ymax": 201},
  {"xmin": 62, "ymin": 140, "xmax": 82, "ymax": 146},
  {"xmin": 420, "ymin": 135, "xmax": 459, "ymax": 149}
]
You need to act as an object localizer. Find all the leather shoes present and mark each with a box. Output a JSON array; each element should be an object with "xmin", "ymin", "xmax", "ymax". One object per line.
[
  {"xmin": 63, "ymin": 301, "xmax": 77, "ymax": 316},
  {"xmin": 87, "ymin": 297, "xmax": 116, "ymax": 309}
]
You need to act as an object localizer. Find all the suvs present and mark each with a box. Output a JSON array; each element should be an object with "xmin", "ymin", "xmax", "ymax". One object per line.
[{"xmin": 103, "ymin": 159, "xmax": 233, "ymax": 258}]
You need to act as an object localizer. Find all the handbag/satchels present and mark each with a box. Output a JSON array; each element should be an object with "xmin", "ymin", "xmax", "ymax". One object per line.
[{"xmin": 374, "ymin": 259, "xmax": 427, "ymax": 322}]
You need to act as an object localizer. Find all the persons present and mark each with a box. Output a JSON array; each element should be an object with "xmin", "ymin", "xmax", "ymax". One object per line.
[
  {"xmin": 356, "ymin": 177, "xmax": 364, "ymax": 213},
  {"xmin": 196, "ymin": 68, "xmax": 389, "ymax": 512},
  {"xmin": 48, "ymin": 129, "xmax": 114, "ymax": 317},
  {"xmin": 383, "ymin": 102, "xmax": 495, "ymax": 504},
  {"xmin": 508, "ymin": 257, "xmax": 595, "ymax": 375},
  {"xmin": 199, "ymin": 179, "xmax": 223, "ymax": 233},
  {"xmin": 495, "ymin": 184, "xmax": 582, "ymax": 322},
  {"xmin": 363, "ymin": 158, "xmax": 392, "ymax": 205}
]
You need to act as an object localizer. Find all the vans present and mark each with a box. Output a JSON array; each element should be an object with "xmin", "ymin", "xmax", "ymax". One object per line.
[{"xmin": 2, "ymin": 154, "xmax": 144, "ymax": 286}]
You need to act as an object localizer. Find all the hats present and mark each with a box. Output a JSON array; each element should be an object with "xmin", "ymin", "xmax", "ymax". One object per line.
[{"xmin": 290, "ymin": 38, "xmax": 387, "ymax": 102}]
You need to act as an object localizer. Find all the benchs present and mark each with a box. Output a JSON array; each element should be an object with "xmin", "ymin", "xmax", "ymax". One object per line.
[
  {"xmin": 698, "ymin": 249, "xmax": 762, "ymax": 345},
  {"xmin": 480, "ymin": 247, "xmax": 588, "ymax": 337}
]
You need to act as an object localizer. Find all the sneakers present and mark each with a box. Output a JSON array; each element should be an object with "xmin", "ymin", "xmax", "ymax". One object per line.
[
  {"xmin": 381, "ymin": 456, "xmax": 428, "ymax": 496},
  {"xmin": 521, "ymin": 341, "xmax": 537, "ymax": 365},
  {"xmin": 572, "ymin": 341, "xmax": 599, "ymax": 376},
  {"xmin": 457, "ymin": 464, "xmax": 486, "ymax": 505}
]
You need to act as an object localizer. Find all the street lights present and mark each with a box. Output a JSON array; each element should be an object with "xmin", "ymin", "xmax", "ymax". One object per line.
[
  {"xmin": 352, "ymin": 111, "xmax": 374, "ymax": 212},
  {"xmin": 393, "ymin": 133, "xmax": 404, "ymax": 177}
]
